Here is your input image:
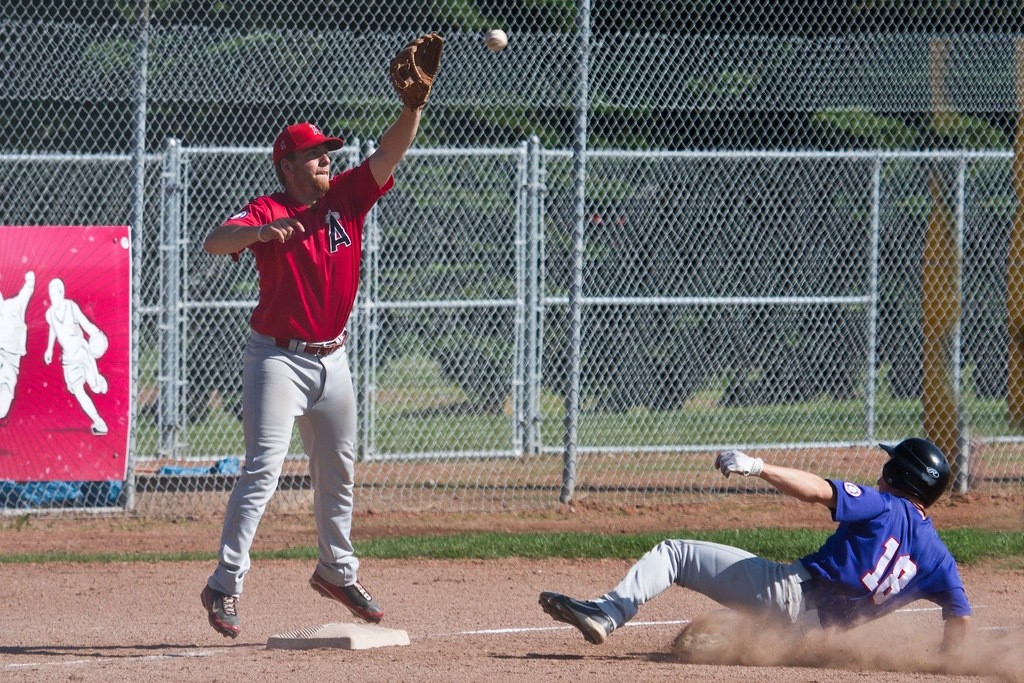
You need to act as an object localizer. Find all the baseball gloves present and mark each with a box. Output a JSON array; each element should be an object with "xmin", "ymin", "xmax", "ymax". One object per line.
[{"xmin": 388, "ymin": 33, "xmax": 446, "ymax": 111}]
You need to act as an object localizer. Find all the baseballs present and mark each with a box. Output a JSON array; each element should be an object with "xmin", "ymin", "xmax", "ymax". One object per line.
[{"xmin": 484, "ymin": 28, "xmax": 508, "ymax": 51}]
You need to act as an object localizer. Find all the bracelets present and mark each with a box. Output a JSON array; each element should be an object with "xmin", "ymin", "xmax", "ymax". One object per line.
[{"xmin": 258, "ymin": 224, "xmax": 268, "ymax": 242}]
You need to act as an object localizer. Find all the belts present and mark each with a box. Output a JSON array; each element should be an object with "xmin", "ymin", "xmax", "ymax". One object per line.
[
  {"xmin": 802, "ymin": 581, "xmax": 815, "ymax": 612},
  {"xmin": 276, "ymin": 333, "xmax": 347, "ymax": 358}
]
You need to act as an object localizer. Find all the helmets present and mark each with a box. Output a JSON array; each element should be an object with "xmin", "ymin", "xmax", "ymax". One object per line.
[{"xmin": 881, "ymin": 438, "xmax": 951, "ymax": 509}]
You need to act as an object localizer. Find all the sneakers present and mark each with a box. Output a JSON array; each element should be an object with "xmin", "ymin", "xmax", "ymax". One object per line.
[
  {"xmin": 308, "ymin": 568, "xmax": 382, "ymax": 624},
  {"xmin": 539, "ymin": 591, "xmax": 614, "ymax": 645},
  {"xmin": 201, "ymin": 584, "xmax": 241, "ymax": 641}
]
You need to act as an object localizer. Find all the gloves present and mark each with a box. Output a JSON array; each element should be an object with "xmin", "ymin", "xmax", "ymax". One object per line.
[{"xmin": 715, "ymin": 449, "xmax": 763, "ymax": 479}]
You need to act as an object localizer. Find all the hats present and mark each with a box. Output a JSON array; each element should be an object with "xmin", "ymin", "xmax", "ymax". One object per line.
[{"xmin": 273, "ymin": 123, "xmax": 344, "ymax": 166}]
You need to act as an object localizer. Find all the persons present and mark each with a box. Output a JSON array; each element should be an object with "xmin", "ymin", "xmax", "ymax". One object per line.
[
  {"xmin": 199, "ymin": 33, "xmax": 446, "ymax": 642},
  {"xmin": 539, "ymin": 437, "xmax": 970, "ymax": 667}
]
